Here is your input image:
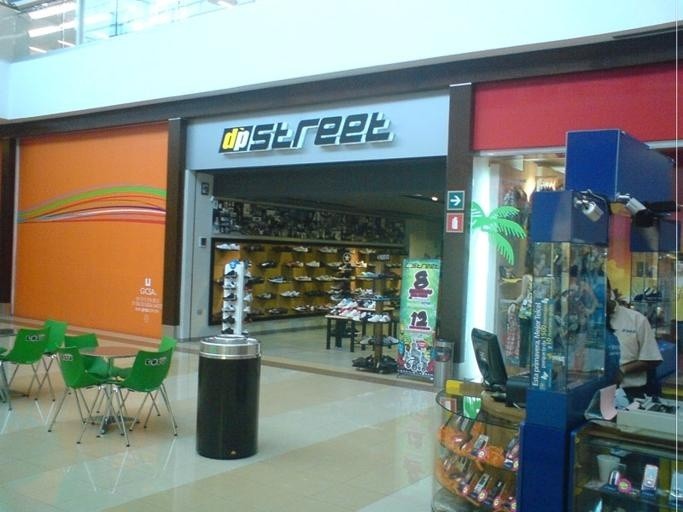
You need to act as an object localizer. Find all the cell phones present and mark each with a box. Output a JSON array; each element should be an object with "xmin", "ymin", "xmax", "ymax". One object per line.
[
  {"xmin": 489, "ymin": 481, "xmax": 505, "ymax": 499},
  {"xmin": 667, "ymin": 469, "xmax": 682, "ymax": 508},
  {"xmin": 607, "ymin": 467, "xmax": 621, "ymax": 487},
  {"xmin": 440, "ymin": 412, "xmax": 489, "ymax": 498},
  {"xmin": 641, "ymin": 464, "xmax": 658, "ymax": 491}
]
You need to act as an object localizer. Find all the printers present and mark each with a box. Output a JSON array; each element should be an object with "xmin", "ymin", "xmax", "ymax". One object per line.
[{"xmin": 617, "ymin": 394, "xmax": 683, "ymax": 444}]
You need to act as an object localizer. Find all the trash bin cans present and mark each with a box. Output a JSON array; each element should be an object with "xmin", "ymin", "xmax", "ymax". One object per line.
[{"xmin": 195, "ymin": 334, "xmax": 261, "ymax": 460}]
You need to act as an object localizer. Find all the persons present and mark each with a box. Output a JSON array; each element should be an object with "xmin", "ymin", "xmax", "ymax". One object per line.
[
  {"xmin": 552, "ymin": 268, "xmax": 600, "ymax": 377},
  {"xmin": 603, "ymin": 277, "xmax": 663, "ymax": 400},
  {"xmin": 506, "ymin": 247, "xmax": 557, "ymax": 370}
]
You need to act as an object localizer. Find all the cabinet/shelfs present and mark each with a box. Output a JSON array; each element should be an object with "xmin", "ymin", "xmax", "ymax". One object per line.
[
  {"xmin": 431, "ymin": 365, "xmax": 683, "ymax": 512},
  {"xmin": 321, "ymin": 252, "xmax": 403, "ymax": 372},
  {"xmin": 207, "ymin": 236, "xmax": 407, "ymax": 325}
]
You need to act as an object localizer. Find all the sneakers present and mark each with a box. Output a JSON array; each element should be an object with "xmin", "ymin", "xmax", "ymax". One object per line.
[
  {"xmin": 222, "ymin": 293, "xmax": 237, "ymax": 301},
  {"xmin": 220, "ymin": 316, "xmax": 234, "ymax": 324},
  {"xmin": 216, "ymin": 242, "xmax": 375, "ymax": 254},
  {"xmin": 242, "ymin": 304, "xmax": 333, "ymax": 314},
  {"xmin": 220, "ymin": 328, "xmax": 233, "ymax": 334},
  {"xmin": 246, "ymin": 260, "xmax": 401, "ymax": 268},
  {"xmin": 359, "ymin": 336, "xmax": 400, "ymax": 346},
  {"xmin": 324, "ymin": 309, "xmax": 391, "ymax": 323},
  {"xmin": 241, "ymin": 288, "xmax": 399, "ymax": 302},
  {"xmin": 351, "ymin": 355, "xmax": 399, "ymax": 374},
  {"xmin": 222, "ymin": 281, "xmax": 236, "ymax": 290},
  {"xmin": 334, "ymin": 298, "xmax": 376, "ymax": 311},
  {"xmin": 223, "ymin": 270, "xmax": 237, "ymax": 278},
  {"xmin": 221, "ymin": 305, "xmax": 234, "ymax": 312},
  {"xmin": 245, "ymin": 273, "xmax": 402, "ymax": 284}
]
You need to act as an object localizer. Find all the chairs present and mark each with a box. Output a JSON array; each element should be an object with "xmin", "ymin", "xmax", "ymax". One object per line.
[{"xmin": 0, "ymin": 319, "xmax": 178, "ymax": 446}]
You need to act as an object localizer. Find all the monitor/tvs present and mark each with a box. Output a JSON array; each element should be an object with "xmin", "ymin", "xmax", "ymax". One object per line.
[{"xmin": 471, "ymin": 328, "xmax": 507, "ymax": 392}]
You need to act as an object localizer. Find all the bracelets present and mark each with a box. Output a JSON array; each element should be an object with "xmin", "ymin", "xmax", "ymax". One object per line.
[{"xmin": 510, "ymin": 302, "xmax": 518, "ymax": 306}]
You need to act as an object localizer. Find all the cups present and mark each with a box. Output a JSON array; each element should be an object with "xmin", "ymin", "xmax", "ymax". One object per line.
[{"xmin": 596, "ymin": 455, "xmax": 627, "ymax": 485}]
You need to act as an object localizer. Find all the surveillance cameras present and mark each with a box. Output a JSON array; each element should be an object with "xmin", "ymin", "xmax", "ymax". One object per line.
[
  {"xmin": 625, "ymin": 196, "xmax": 647, "ymax": 218},
  {"xmin": 580, "ymin": 198, "xmax": 604, "ymax": 222}
]
